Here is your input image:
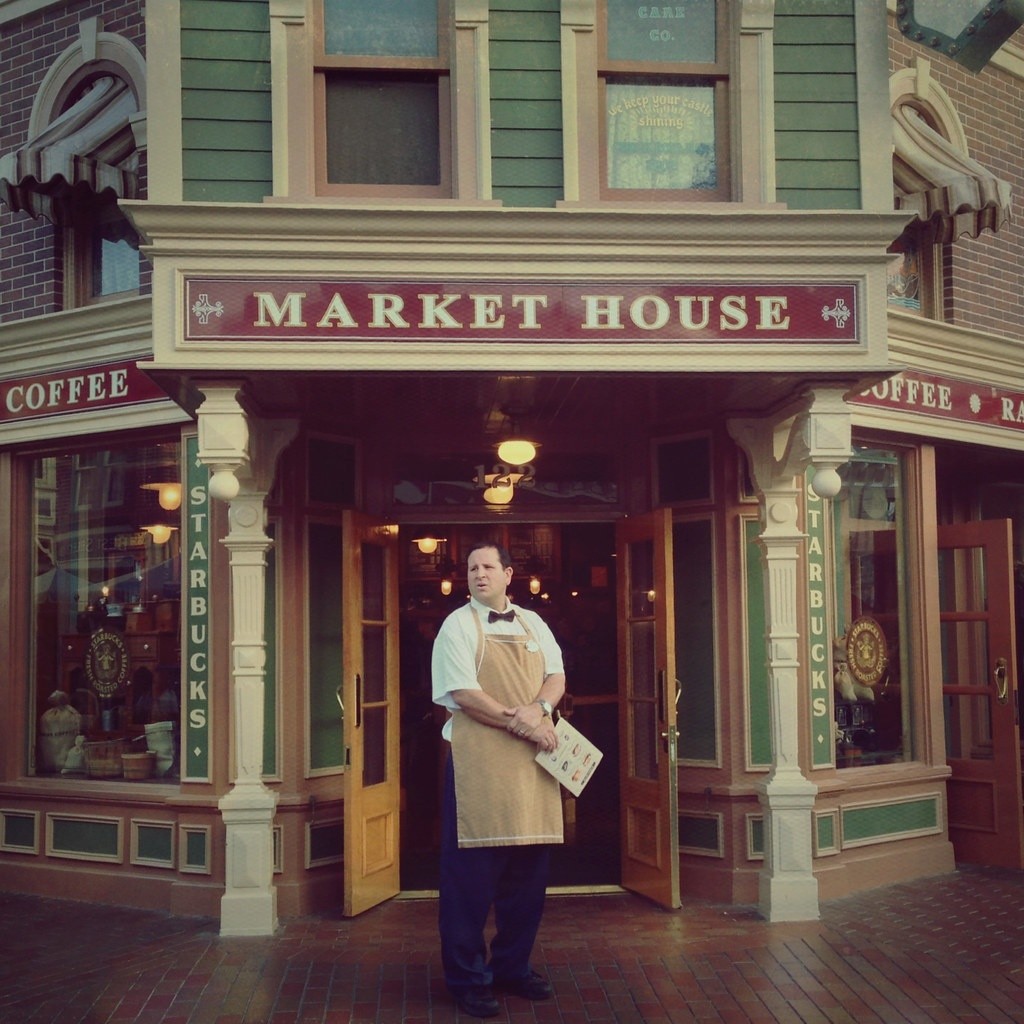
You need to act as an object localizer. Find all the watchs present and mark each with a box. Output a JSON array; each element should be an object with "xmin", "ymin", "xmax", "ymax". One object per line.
[{"xmin": 534, "ymin": 699, "xmax": 552, "ymax": 715}]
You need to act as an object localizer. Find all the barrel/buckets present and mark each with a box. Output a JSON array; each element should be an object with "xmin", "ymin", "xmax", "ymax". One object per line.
[
  {"xmin": 839, "ymin": 740, "xmax": 878, "ymax": 768},
  {"xmin": 82, "ymin": 737, "xmax": 127, "ymax": 779},
  {"xmin": 120, "ymin": 750, "xmax": 158, "ymax": 780}
]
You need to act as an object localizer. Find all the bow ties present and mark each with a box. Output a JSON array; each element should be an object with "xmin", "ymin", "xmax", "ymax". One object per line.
[{"xmin": 488, "ymin": 609, "xmax": 515, "ymax": 623}]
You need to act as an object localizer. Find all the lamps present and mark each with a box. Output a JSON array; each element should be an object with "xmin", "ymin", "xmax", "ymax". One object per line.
[
  {"xmin": 138, "ymin": 522, "xmax": 179, "ymax": 543},
  {"xmin": 441, "ymin": 576, "xmax": 454, "ymax": 595},
  {"xmin": 528, "ymin": 574, "xmax": 543, "ymax": 594},
  {"xmin": 410, "ymin": 525, "xmax": 447, "ymax": 553},
  {"xmin": 138, "ymin": 478, "xmax": 181, "ymax": 510},
  {"xmin": 492, "ymin": 417, "xmax": 542, "ymax": 465}
]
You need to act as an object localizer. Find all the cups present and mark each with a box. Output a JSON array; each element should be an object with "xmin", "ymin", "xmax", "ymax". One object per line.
[
  {"xmin": 836, "ymin": 706, "xmax": 846, "ymax": 727},
  {"xmin": 852, "ymin": 705, "xmax": 864, "ymax": 726}
]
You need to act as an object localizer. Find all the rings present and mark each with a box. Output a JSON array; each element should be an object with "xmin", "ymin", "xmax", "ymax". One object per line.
[{"xmin": 520, "ymin": 729, "xmax": 525, "ymax": 735}]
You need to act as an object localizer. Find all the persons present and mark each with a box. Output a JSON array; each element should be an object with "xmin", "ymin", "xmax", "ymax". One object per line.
[{"xmin": 431, "ymin": 547, "xmax": 565, "ymax": 1017}]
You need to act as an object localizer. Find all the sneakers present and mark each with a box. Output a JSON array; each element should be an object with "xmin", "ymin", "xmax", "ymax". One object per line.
[
  {"xmin": 462, "ymin": 985, "xmax": 499, "ymax": 1017},
  {"xmin": 486, "ymin": 967, "xmax": 552, "ymax": 999}
]
